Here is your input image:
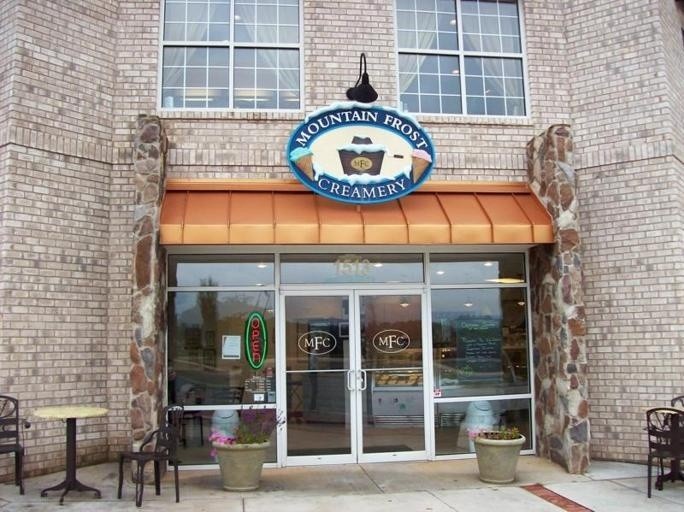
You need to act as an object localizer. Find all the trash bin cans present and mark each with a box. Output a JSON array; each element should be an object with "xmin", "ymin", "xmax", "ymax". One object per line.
[
  {"xmin": 210, "ymin": 408, "xmax": 240, "ymax": 463},
  {"xmin": 466, "ymin": 400, "xmax": 493, "ymax": 453}
]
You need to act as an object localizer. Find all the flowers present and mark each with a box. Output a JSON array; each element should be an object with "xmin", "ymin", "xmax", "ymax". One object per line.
[
  {"xmin": 468, "ymin": 424, "xmax": 521, "ymax": 440},
  {"xmin": 208, "ymin": 403, "xmax": 286, "ymax": 458}
]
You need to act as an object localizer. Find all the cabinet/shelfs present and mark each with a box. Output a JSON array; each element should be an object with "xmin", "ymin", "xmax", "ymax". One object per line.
[{"xmin": 370, "ymin": 345, "xmax": 528, "ymax": 428}]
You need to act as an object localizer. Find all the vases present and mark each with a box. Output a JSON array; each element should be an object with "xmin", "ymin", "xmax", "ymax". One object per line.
[
  {"xmin": 212, "ymin": 436, "xmax": 271, "ymax": 492},
  {"xmin": 471, "ymin": 430, "xmax": 527, "ymax": 484}
]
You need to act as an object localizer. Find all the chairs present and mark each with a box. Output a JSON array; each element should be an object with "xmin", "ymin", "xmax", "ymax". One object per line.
[
  {"xmin": 117, "ymin": 402, "xmax": 185, "ymax": 508},
  {"xmin": 646, "ymin": 397, "xmax": 684, "ymax": 498},
  {"xmin": 0, "ymin": 395, "xmax": 31, "ymax": 495}
]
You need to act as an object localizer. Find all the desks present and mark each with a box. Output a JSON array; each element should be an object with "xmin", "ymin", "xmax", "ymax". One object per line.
[{"xmin": 32, "ymin": 406, "xmax": 110, "ymax": 506}]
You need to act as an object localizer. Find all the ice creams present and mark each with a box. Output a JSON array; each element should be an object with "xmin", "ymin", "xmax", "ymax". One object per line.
[
  {"xmin": 411, "ymin": 149, "xmax": 432, "ymax": 183},
  {"xmin": 289, "ymin": 147, "xmax": 316, "ymax": 182}
]
